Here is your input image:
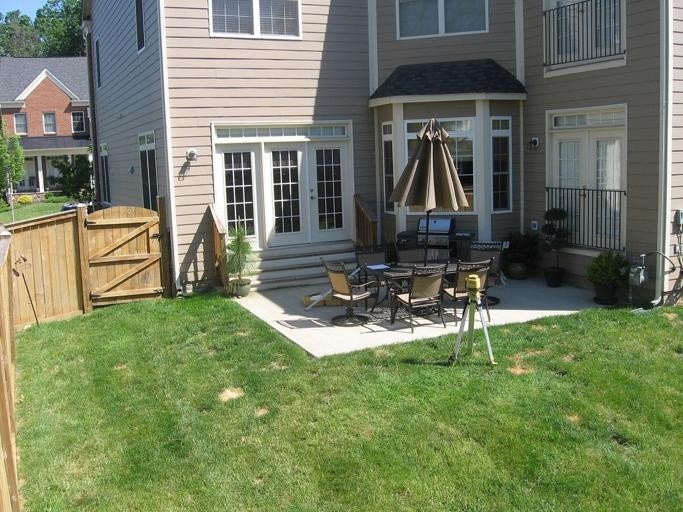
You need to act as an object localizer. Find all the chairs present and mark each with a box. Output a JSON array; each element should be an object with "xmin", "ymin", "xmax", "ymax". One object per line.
[{"xmin": 319, "ymin": 239, "xmax": 502, "ymax": 334}]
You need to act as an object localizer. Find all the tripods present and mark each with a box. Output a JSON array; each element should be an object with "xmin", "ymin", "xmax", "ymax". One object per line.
[{"xmin": 448, "ymin": 275, "xmax": 498, "ymax": 365}]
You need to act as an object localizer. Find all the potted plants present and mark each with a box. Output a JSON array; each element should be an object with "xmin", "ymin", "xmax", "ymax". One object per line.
[
  {"xmin": 502, "ymin": 207, "xmax": 569, "ymax": 287},
  {"xmin": 218, "ymin": 223, "xmax": 261, "ymax": 299},
  {"xmin": 586, "ymin": 248, "xmax": 631, "ymax": 306}
]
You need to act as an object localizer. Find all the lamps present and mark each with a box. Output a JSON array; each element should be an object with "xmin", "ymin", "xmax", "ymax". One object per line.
[{"xmin": 527, "ymin": 136, "xmax": 540, "ymax": 151}]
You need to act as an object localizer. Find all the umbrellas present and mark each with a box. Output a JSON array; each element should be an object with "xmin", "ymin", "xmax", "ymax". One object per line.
[{"xmin": 389, "ymin": 117, "xmax": 470, "ymax": 268}]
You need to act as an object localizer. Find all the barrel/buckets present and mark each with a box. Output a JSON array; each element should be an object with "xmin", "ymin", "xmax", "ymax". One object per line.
[{"xmin": 543, "ymin": 268, "xmax": 568, "ymax": 288}]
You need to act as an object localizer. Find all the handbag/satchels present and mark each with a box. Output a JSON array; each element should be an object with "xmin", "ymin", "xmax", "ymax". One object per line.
[
  {"xmin": 499, "ymin": 250, "xmax": 511, "ymax": 271},
  {"xmin": 510, "ymin": 236, "xmax": 530, "ymax": 262}
]
